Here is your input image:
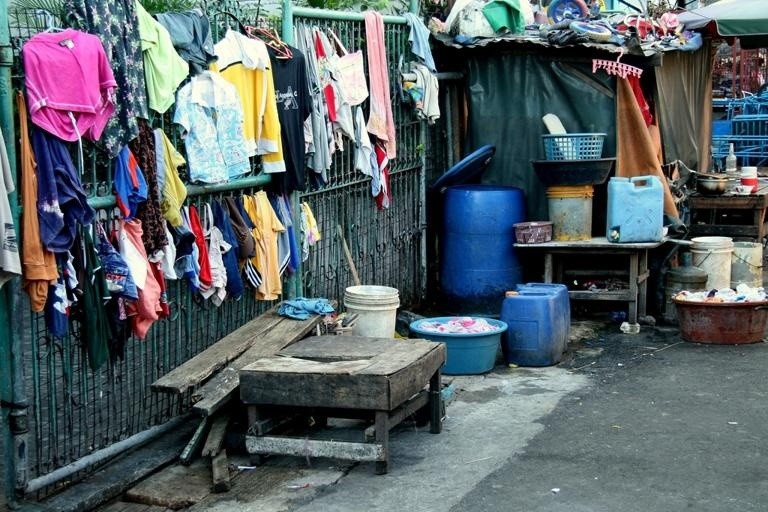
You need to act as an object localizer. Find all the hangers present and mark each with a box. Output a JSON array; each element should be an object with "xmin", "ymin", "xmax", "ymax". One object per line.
[
  {"xmin": 34, "ymin": 7, "xmax": 69, "ymax": 34},
  {"xmin": 196, "ymin": 17, "xmax": 292, "ymax": 83}
]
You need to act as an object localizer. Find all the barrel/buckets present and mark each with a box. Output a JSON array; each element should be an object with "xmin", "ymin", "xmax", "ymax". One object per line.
[
  {"xmin": 546, "ymin": 185, "xmax": 594, "ymax": 242},
  {"xmin": 730, "ymin": 242, "xmax": 763, "ymax": 290},
  {"xmin": 343, "ymin": 284, "xmax": 400, "ymax": 340},
  {"xmin": 691, "ymin": 237, "xmax": 734, "ymax": 291},
  {"xmin": 605, "ymin": 177, "xmax": 664, "ymax": 243},
  {"xmin": 500, "ymin": 281, "xmax": 571, "ymax": 367},
  {"xmin": 445, "ymin": 187, "xmax": 524, "ymax": 317}
]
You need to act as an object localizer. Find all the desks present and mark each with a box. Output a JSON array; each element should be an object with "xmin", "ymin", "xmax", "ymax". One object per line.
[
  {"xmin": 239, "ymin": 336, "xmax": 447, "ymax": 476},
  {"xmin": 686, "ymin": 175, "xmax": 768, "ymax": 244},
  {"xmin": 511, "ymin": 236, "xmax": 671, "ymax": 325}
]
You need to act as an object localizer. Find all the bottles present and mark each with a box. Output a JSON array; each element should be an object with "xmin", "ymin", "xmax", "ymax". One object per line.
[
  {"xmin": 740, "ymin": 166, "xmax": 759, "ymax": 193},
  {"xmin": 726, "ymin": 142, "xmax": 737, "ymax": 171}
]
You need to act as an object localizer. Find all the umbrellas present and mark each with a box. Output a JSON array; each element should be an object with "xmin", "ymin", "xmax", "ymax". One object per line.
[{"xmin": 679, "ymin": 0, "xmax": 768, "ymax": 50}]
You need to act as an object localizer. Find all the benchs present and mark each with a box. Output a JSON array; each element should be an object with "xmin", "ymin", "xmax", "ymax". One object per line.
[{"xmin": 149, "ymin": 296, "xmax": 337, "ymax": 463}]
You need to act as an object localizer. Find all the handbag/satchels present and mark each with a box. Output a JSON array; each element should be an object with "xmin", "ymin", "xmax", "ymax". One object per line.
[{"xmin": 332, "ymin": 49, "xmax": 369, "ymax": 106}]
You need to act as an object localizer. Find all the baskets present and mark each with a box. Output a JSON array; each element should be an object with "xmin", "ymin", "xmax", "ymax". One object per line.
[{"xmin": 541, "ymin": 132, "xmax": 607, "ymax": 160}]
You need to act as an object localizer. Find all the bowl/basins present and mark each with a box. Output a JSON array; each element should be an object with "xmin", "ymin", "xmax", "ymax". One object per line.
[
  {"xmin": 411, "ymin": 315, "xmax": 507, "ymax": 377},
  {"xmin": 512, "ymin": 220, "xmax": 554, "ymax": 242},
  {"xmin": 529, "ymin": 157, "xmax": 617, "ymax": 187},
  {"xmin": 671, "ymin": 294, "xmax": 768, "ymax": 343},
  {"xmin": 736, "ymin": 185, "xmax": 754, "ymax": 195}
]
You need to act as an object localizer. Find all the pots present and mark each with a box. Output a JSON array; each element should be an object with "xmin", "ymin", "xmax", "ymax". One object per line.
[{"xmin": 697, "ymin": 177, "xmax": 728, "ymax": 195}]
